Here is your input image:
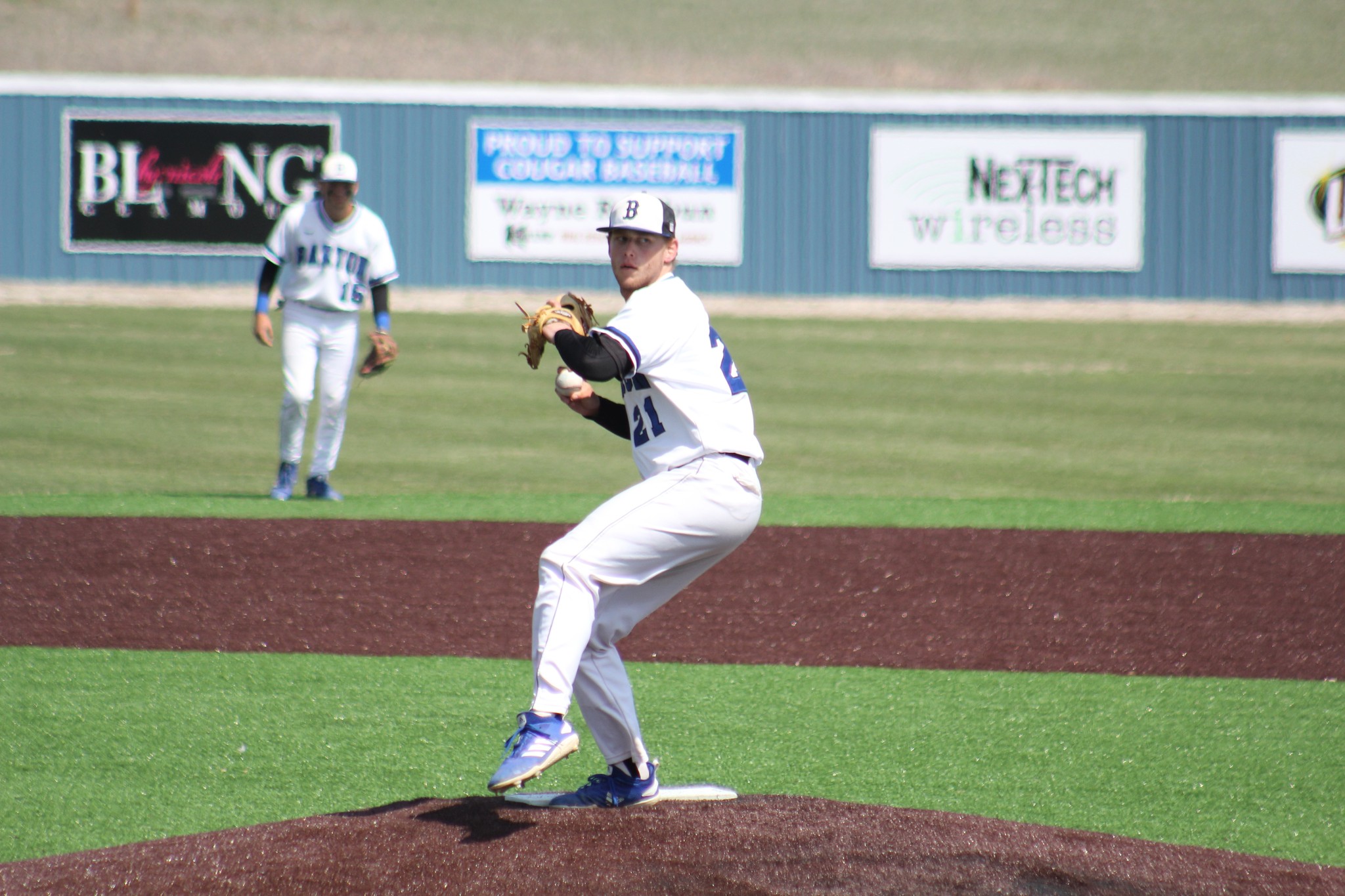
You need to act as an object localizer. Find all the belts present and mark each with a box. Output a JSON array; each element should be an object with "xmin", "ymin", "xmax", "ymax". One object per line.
[{"xmin": 721, "ymin": 452, "xmax": 749, "ymax": 464}]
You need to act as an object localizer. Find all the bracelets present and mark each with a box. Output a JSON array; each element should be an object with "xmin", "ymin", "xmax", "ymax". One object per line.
[
  {"xmin": 255, "ymin": 296, "xmax": 269, "ymax": 313},
  {"xmin": 375, "ymin": 313, "xmax": 390, "ymax": 331}
]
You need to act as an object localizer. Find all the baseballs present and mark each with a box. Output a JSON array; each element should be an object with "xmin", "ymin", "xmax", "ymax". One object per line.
[{"xmin": 555, "ymin": 367, "xmax": 584, "ymax": 397}]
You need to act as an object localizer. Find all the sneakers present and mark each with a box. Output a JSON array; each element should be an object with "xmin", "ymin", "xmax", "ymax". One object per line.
[
  {"xmin": 307, "ymin": 476, "xmax": 342, "ymax": 502},
  {"xmin": 270, "ymin": 461, "xmax": 299, "ymax": 501},
  {"xmin": 487, "ymin": 713, "xmax": 579, "ymax": 793},
  {"xmin": 548, "ymin": 760, "xmax": 660, "ymax": 811}
]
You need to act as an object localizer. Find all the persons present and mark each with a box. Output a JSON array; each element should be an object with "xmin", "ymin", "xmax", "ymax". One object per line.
[
  {"xmin": 255, "ymin": 152, "xmax": 399, "ymax": 508},
  {"xmin": 487, "ymin": 191, "xmax": 764, "ymax": 811}
]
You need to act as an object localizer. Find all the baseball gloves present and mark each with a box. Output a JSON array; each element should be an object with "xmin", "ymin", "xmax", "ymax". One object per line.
[
  {"xmin": 360, "ymin": 329, "xmax": 397, "ymax": 379},
  {"xmin": 514, "ymin": 290, "xmax": 599, "ymax": 370}
]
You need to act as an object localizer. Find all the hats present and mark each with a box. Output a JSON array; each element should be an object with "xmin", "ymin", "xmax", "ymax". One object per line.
[
  {"xmin": 596, "ymin": 193, "xmax": 675, "ymax": 238},
  {"xmin": 319, "ymin": 151, "xmax": 358, "ymax": 182}
]
